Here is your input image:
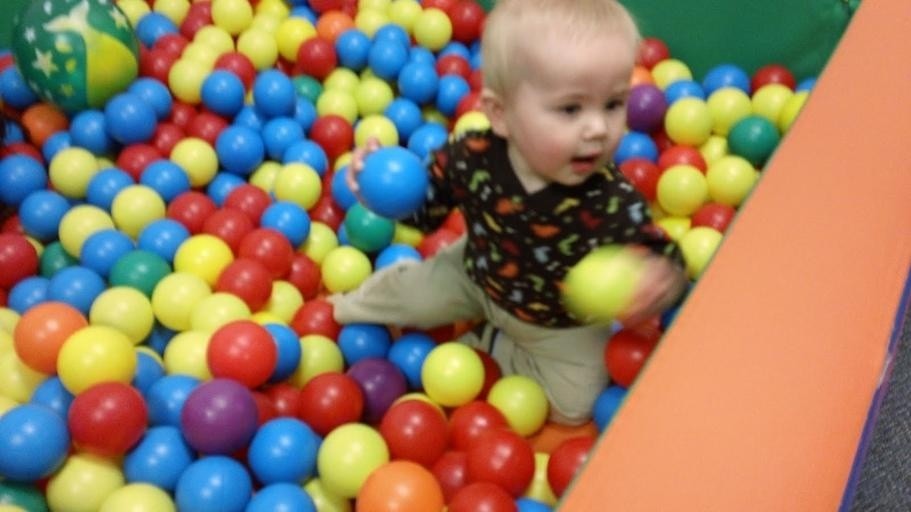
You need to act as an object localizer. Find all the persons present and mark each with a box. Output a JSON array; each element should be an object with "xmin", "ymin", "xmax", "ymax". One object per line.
[{"xmin": 325, "ymin": 1, "xmax": 692, "ymax": 427}]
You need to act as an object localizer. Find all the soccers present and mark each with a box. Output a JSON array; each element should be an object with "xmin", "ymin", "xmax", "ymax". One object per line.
[{"xmin": 11, "ymin": 0, "xmax": 138, "ymax": 113}]
